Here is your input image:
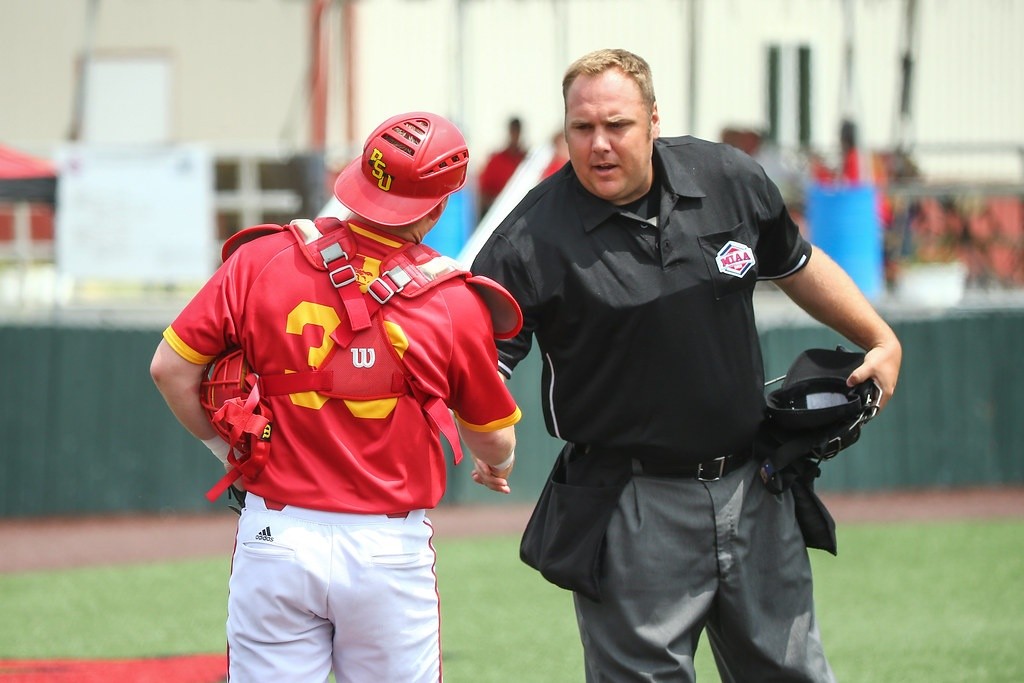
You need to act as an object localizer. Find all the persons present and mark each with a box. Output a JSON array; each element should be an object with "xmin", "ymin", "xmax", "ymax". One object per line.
[
  {"xmin": 150, "ymin": 112, "xmax": 523, "ymax": 683},
  {"xmin": 832, "ymin": 121, "xmax": 893, "ymax": 232},
  {"xmin": 469, "ymin": 49, "xmax": 903, "ymax": 683},
  {"xmin": 479, "ymin": 118, "xmax": 530, "ymax": 196}
]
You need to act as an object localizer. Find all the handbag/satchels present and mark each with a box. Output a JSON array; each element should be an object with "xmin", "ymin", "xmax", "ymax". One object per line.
[
  {"xmin": 519, "ymin": 442, "xmax": 631, "ymax": 605},
  {"xmin": 784, "ymin": 459, "xmax": 838, "ymax": 556}
]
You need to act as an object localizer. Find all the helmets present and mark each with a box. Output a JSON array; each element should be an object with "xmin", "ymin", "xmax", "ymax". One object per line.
[
  {"xmin": 334, "ymin": 112, "xmax": 469, "ymax": 228},
  {"xmin": 200, "ymin": 347, "xmax": 251, "ymax": 454}
]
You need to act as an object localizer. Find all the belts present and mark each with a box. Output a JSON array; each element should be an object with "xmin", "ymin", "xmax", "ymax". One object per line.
[
  {"xmin": 262, "ymin": 497, "xmax": 409, "ymax": 518},
  {"xmin": 628, "ymin": 445, "xmax": 760, "ymax": 483}
]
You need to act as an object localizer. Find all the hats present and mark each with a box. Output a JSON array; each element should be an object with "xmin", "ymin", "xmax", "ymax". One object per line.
[{"xmin": 766, "ymin": 348, "xmax": 872, "ymax": 453}]
